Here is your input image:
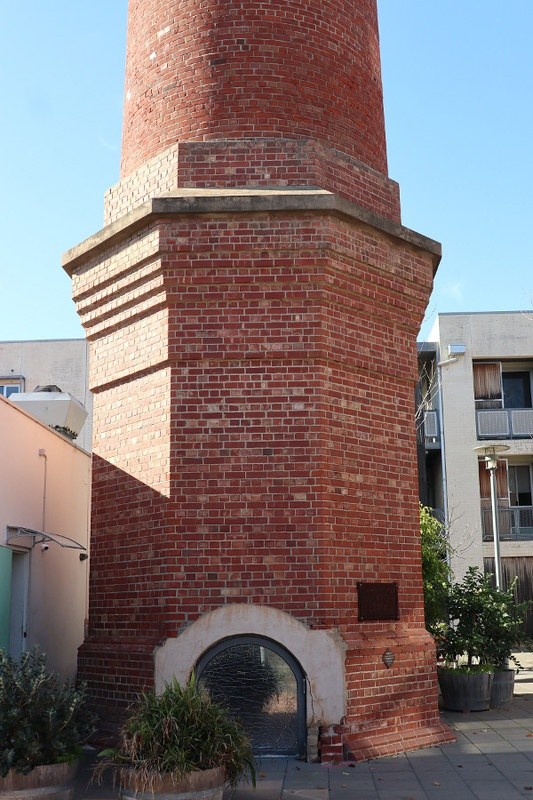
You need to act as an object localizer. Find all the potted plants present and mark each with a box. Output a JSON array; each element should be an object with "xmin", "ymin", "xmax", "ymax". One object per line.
[
  {"xmin": 431, "ymin": 566, "xmax": 533, "ymax": 711},
  {"xmin": 0, "ymin": 645, "xmax": 101, "ymax": 800},
  {"xmin": 88, "ymin": 670, "xmax": 258, "ymax": 800}
]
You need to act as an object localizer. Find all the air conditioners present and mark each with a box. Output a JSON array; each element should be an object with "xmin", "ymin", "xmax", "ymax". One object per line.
[{"xmin": 422, "ymin": 410, "xmax": 441, "ymax": 450}]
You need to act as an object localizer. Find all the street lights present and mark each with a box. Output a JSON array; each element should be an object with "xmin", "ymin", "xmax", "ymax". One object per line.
[{"xmin": 474, "ymin": 444, "xmax": 511, "ymax": 591}]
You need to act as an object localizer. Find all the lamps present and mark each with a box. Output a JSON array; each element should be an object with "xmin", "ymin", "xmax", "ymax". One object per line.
[{"xmin": 449, "ymin": 344, "xmax": 466, "ymax": 355}]
